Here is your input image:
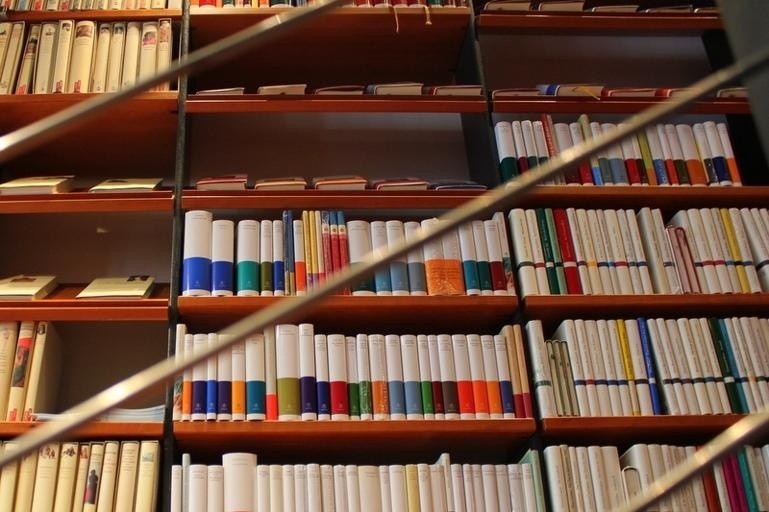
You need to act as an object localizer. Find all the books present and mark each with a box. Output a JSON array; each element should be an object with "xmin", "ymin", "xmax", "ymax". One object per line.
[
  {"xmin": 482, "ymin": 0, "xmax": 533, "ymax": 10},
  {"xmin": 184, "ymin": 210, "xmax": 521, "ymax": 299},
  {"xmin": 424, "ymin": 84, "xmax": 485, "ymax": 96},
  {"xmin": 341, "ymin": 0, "xmax": 471, "ymax": 8},
  {"xmin": 170, "ymin": 448, "xmax": 547, "ymax": 512},
  {"xmin": 196, "ymin": 87, "xmax": 246, "ymax": 96},
  {"xmin": 0, "ymin": 0, "xmax": 183, "ymax": 9},
  {"xmin": 602, "ymin": 87, "xmax": 658, "ymax": 96},
  {"xmin": 1, "ymin": 174, "xmax": 80, "ymax": 195},
  {"xmin": 311, "ymin": 174, "xmax": 366, "ymax": 190},
  {"xmin": 495, "ymin": 121, "xmax": 744, "ymax": 187},
  {"xmin": 77, "ymin": 276, "xmax": 156, "ymax": 301},
  {"xmin": 540, "ymin": 2, "xmax": 586, "ymax": 12},
  {"xmin": 536, "ymin": 83, "xmax": 606, "ymax": 96},
  {"xmin": 0, "ymin": 322, "xmax": 64, "ymax": 423},
  {"xmin": 254, "ymin": 176, "xmax": 307, "ymax": 191},
  {"xmin": 370, "ymin": 177, "xmax": 429, "ymax": 191},
  {"xmin": 196, "ymin": 174, "xmax": 249, "ymax": 190},
  {"xmin": 509, "ymin": 207, "xmax": 769, "ymax": 295},
  {"xmin": 1, "ymin": 20, "xmax": 172, "ymax": 94},
  {"xmin": 643, "ymin": 4, "xmax": 694, "ymax": 14},
  {"xmin": 694, "ymin": 7, "xmax": 723, "ymax": 14},
  {"xmin": 431, "ymin": 179, "xmax": 488, "ymax": 191},
  {"xmin": 525, "ymin": 319, "xmax": 769, "ymax": 419},
  {"xmin": 488, "ymin": 88, "xmax": 542, "ymax": 95},
  {"xmin": 657, "ymin": 88, "xmax": 700, "ymax": 97},
  {"xmin": 189, "ymin": 0, "xmax": 315, "ymax": 8},
  {"xmin": 543, "ymin": 444, "xmax": 769, "ymax": 512},
  {"xmin": 88, "ymin": 404, "xmax": 168, "ymax": 424},
  {"xmin": 709, "ymin": 87, "xmax": 750, "ymax": 97},
  {"xmin": 174, "ymin": 323, "xmax": 540, "ymax": 420},
  {"xmin": 88, "ymin": 177, "xmax": 165, "ymax": 193},
  {"xmin": 365, "ymin": 83, "xmax": 422, "ymax": 94},
  {"xmin": 259, "ymin": 84, "xmax": 308, "ymax": 95},
  {"xmin": 590, "ymin": 4, "xmax": 640, "ymax": 13},
  {"xmin": 0, "ymin": 438, "xmax": 163, "ymax": 512},
  {"xmin": 1, "ymin": 274, "xmax": 59, "ymax": 301},
  {"xmin": 315, "ymin": 84, "xmax": 363, "ymax": 95}
]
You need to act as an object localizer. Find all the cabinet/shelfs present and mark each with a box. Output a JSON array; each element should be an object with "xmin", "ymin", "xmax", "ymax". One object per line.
[{"xmin": 0, "ymin": 1, "xmax": 769, "ymax": 512}]
